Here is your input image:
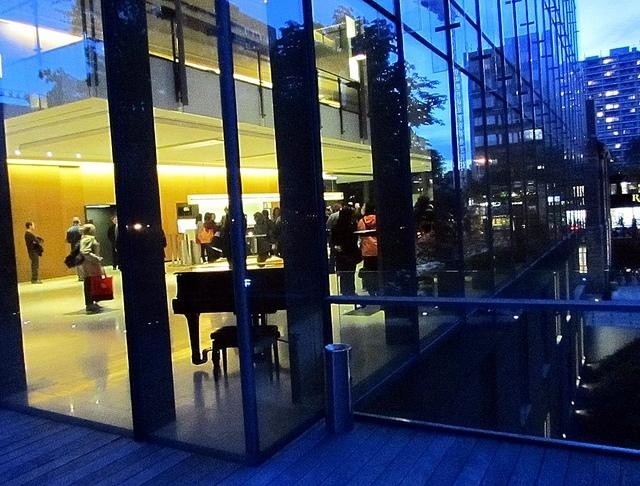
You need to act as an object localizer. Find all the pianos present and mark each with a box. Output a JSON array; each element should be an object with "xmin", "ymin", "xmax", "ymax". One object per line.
[{"xmin": 173, "ymin": 254, "xmax": 287, "ymax": 365}]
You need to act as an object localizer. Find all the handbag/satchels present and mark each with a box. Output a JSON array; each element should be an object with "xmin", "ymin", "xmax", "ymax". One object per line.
[
  {"xmin": 89, "ymin": 274, "xmax": 114, "ymax": 301},
  {"xmin": 63, "ymin": 252, "xmax": 85, "ymax": 269}
]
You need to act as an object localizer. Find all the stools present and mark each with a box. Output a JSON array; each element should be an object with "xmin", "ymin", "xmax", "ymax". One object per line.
[{"xmin": 209, "ymin": 322, "xmax": 282, "ymax": 387}]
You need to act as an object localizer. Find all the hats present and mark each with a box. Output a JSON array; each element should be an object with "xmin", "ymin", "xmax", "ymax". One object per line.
[
  {"xmin": 72, "ymin": 216, "xmax": 80, "ymax": 222},
  {"xmin": 81, "ymin": 224, "xmax": 96, "ymax": 235}
]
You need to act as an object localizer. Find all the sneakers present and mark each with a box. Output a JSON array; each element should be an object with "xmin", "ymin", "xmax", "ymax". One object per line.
[{"xmin": 86, "ymin": 303, "xmax": 103, "ymax": 314}]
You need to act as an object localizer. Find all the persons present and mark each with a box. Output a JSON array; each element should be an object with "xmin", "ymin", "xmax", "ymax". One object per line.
[
  {"xmin": 67, "ymin": 216, "xmax": 103, "ymax": 313},
  {"xmin": 24, "ymin": 221, "xmax": 44, "ymax": 282},
  {"xmin": 324, "ymin": 196, "xmax": 437, "ymax": 296},
  {"xmin": 196, "ymin": 207, "xmax": 282, "ymax": 262},
  {"xmin": 107, "ymin": 216, "xmax": 121, "ymax": 270}
]
